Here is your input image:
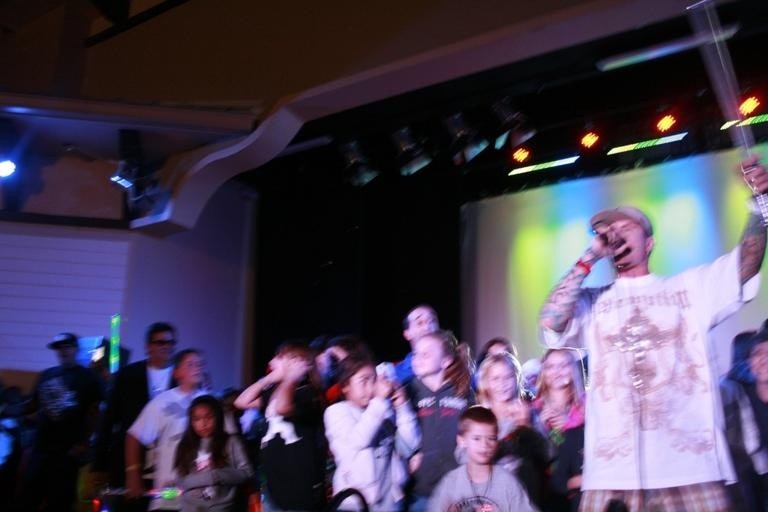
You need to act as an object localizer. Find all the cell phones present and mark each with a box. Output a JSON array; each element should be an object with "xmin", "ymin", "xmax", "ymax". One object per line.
[{"xmin": 376, "ymin": 362, "xmax": 397, "ymax": 381}]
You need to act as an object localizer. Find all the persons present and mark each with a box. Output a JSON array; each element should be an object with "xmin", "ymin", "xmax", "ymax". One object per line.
[
  {"xmin": 2, "ymin": 308, "xmax": 587, "ymax": 511},
  {"xmin": 538, "ymin": 155, "xmax": 768, "ymax": 512}
]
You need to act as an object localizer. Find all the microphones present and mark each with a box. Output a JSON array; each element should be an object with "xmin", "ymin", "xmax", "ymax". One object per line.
[{"xmin": 594, "ymin": 221, "xmax": 630, "ymax": 258}]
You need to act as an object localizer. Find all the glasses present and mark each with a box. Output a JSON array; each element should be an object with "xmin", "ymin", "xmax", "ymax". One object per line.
[{"xmin": 149, "ymin": 338, "xmax": 176, "ymax": 346}]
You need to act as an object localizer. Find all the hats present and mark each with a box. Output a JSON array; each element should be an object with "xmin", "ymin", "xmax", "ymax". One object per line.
[
  {"xmin": 46, "ymin": 333, "xmax": 78, "ymax": 350},
  {"xmin": 590, "ymin": 205, "xmax": 653, "ymax": 237}
]
